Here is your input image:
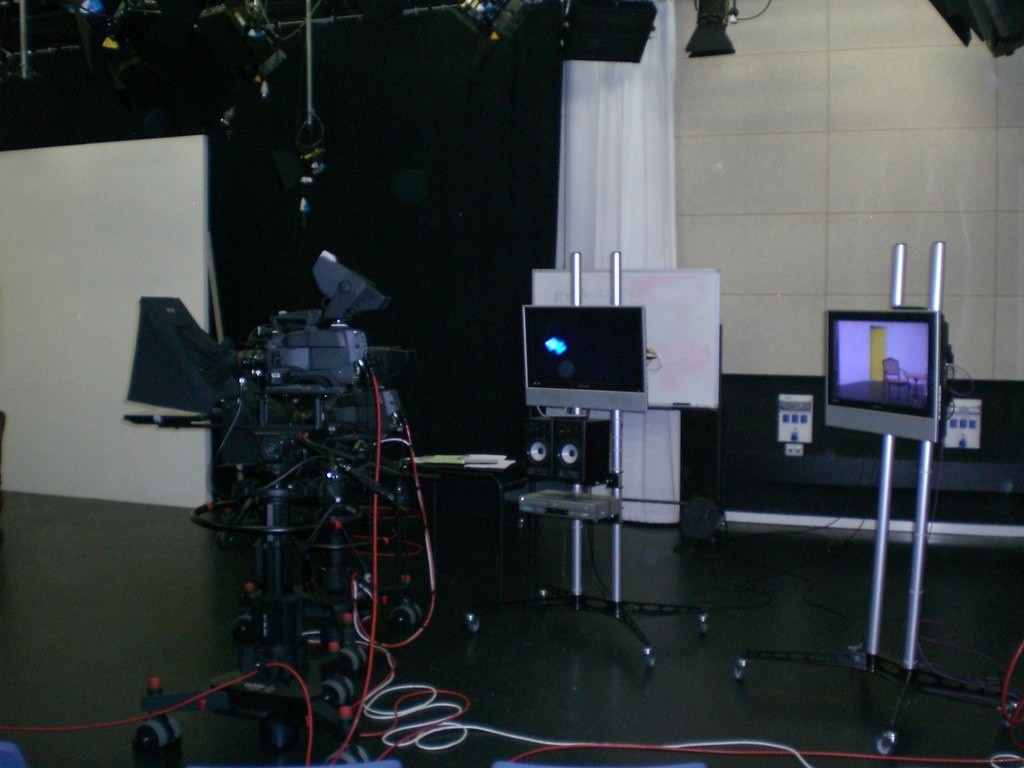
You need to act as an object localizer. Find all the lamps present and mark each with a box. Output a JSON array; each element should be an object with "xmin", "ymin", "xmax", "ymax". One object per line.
[
  {"xmin": 101, "ymin": 0, "xmax": 140, "ymax": 49},
  {"xmin": 220, "ymin": 105, "xmax": 239, "ymax": 127},
  {"xmin": 254, "ymin": 50, "xmax": 287, "ymax": 83},
  {"xmin": 685, "ymin": 0, "xmax": 736, "ymax": 59},
  {"xmin": 300, "ymin": 146, "xmax": 325, "ymax": 164}
]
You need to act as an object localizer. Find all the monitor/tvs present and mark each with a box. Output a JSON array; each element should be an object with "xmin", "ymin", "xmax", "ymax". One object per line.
[
  {"xmin": 522, "ymin": 305, "xmax": 648, "ymax": 414},
  {"xmin": 825, "ymin": 310, "xmax": 943, "ymax": 442}
]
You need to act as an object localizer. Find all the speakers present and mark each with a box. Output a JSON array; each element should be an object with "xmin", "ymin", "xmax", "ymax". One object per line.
[{"xmin": 530, "ymin": 420, "xmax": 612, "ymax": 486}]
